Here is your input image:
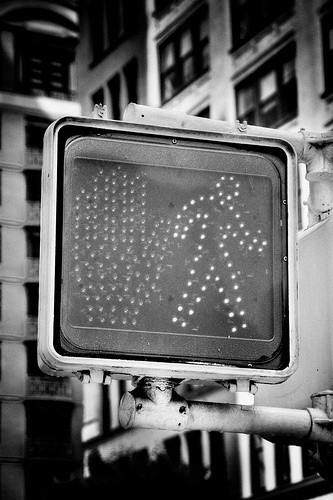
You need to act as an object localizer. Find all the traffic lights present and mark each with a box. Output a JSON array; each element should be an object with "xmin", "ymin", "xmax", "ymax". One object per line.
[{"xmin": 34, "ymin": 114, "xmax": 301, "ymax": 387}]
